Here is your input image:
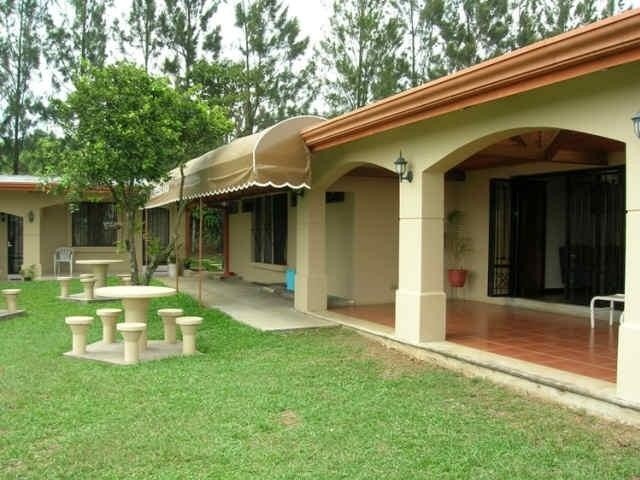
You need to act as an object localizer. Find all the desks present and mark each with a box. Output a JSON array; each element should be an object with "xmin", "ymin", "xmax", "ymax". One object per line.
[
  {"xmin": 75, "ymin": 259, "xmax": 125, "ymax": 299},
  {"xmin": 95, "ymin": 285, "xmax": 176, "ymax": 354}
]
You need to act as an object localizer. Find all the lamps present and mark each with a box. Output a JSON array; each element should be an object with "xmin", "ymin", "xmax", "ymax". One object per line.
[
  {"xmin": 631, "ymin": 108, "xmax": 640, "ymax": 137},
  {"xmin": 392, "ymin": 149, "xmax": 413, "ymax": 183}
]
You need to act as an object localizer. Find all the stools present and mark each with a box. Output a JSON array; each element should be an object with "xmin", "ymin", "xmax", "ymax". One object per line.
[
  {"xmin": 53, "ymin": 274, "xmax": 133, "ymax": 302},
  {"xmin": 0, "ymin": 289, "xmax": 21, "ymax": 313},
  {"xmin": 64, "ymin": 308, "xmax": 203, "ymax": 362}
]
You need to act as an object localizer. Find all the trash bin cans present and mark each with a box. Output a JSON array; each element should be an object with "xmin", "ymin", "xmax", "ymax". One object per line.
[{"xmin": 287, "ymin": 269, "xmax": 295, "ymax": 290}]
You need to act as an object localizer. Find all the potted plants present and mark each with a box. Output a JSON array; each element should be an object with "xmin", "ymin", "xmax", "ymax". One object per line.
[{"xmin": 444, "ymin": 207, "xmax": 471, "ymax": 287}]
